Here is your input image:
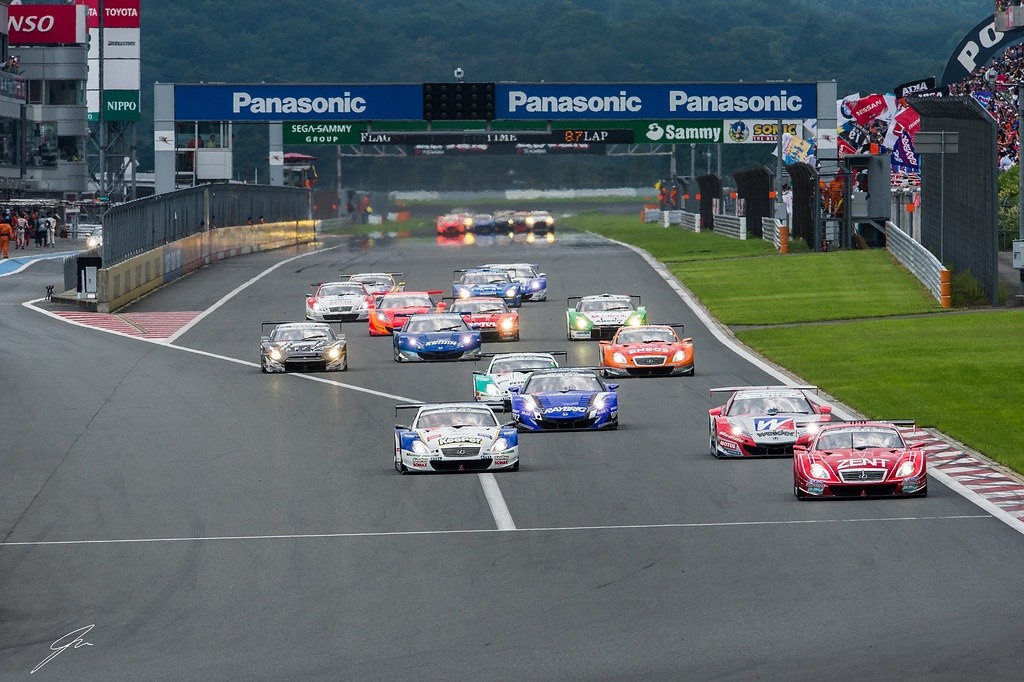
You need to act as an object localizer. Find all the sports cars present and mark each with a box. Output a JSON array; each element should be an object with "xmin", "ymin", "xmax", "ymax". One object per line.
[
  {"xmin": 392, "ymin": 311, "xmax": 483, "ymax": 364},
  {"xmin": 704, "ymin": 384, "xmax": 832, "ymax": 459},
  {"xmin": 339, "ymin": 272, "xmax": 406, "ymax": 301},
  {"xmin": 565, "ymin": 294, "xmax": 648, "ymax": 341},
  {"xmin": 366, "ymin": 290, "xmax": 446, "ymax": 337},
  {"xmin": 440, "ymin": 296, "xmax": 520, "ymax": 343},
  {"xmin": 434, "ymin": 208, "xmax": 556, "ymax": 239},
  {"xmin": 792, "ymin": 418, "xmax": 928, "ymax": 500},
  {"xmin": 452, "ymin": 262, "xmax": 547, "ymax": 308},
  {"xmin": 259, "ymin": 318, "xmax": 349, "ymax": 375},
  {"xmin": 594, "ymin": 322, "xmax": 696, "ymax": 379},
  {"xmin": 305, "ymin": 282, "xmax": 378, "ymax": 324},
  {"xmin": 472, "ymin": 350, "xmax": 568, "ymax": 411},
  {"xmin": 507, "ymin": 365, "xmax": 619, "ymax": 433},
  {"xmin": 391, "ymin": 399, "xmax": 522, "ymax": 475}
]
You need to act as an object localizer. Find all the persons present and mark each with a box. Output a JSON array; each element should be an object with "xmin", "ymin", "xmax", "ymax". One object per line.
[
  {"xmin": 871, "ymin": 0, "xmax": 1024, "ymax": 188},
  {"xmin": 186, "ymin": 133, "xmax": 218, "ymax": 172},
  {"xmin": 0, "ymin": 56, "xmax": 82, "ymax": 166},
  {"xmin": 202, "ymin": 216, "xmax": 265, "ymax": 231},
  {"xmin": 814, "ymin": 171, "xmax": 845, "ymax": 217},
  {"xmin": 0, "ymin": 208, "xmax": 58, "ymax": 259}
]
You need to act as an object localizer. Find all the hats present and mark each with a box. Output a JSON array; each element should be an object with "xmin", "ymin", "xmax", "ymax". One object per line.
[
  {"xmin": 21, "ymin": 212, "xmax": 27, "ymax": 216},
  {"xmin": 41, "ymin": 213, "xmax": 46, "ymax": 217}
]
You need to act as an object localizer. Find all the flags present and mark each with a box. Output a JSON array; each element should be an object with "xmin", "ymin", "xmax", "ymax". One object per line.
[
  {"xmin": 971, "ymin": 91, "xmax": 993, "ymax": 105},
  {"xmin": 768, "ymin": 90, "xmax": 922, "ymax": 178}
]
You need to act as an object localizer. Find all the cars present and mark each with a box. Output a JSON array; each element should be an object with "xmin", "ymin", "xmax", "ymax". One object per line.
[{"xmin": 85, "ymin": 225, "xmax": 103, "ymax": 251}]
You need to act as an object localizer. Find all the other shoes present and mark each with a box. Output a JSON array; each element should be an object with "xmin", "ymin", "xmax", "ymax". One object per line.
[
  {"xmin": 15, "ymin": 247, "xmax": 18, "ymax": 249},
  {"xmin": 22, "ymin": 246, "xmax": 24, "ymax": 249},
  {"xmin": 4, "ymin": 256, "xmax": 8, "ymax": 258},
  {"xmin": 47, "ymin": 245, "xmax": 50, "ymax": 247},
  {"xmin": 53, "ymin": 244, "xmax": 54, "ymax": 246}
]
